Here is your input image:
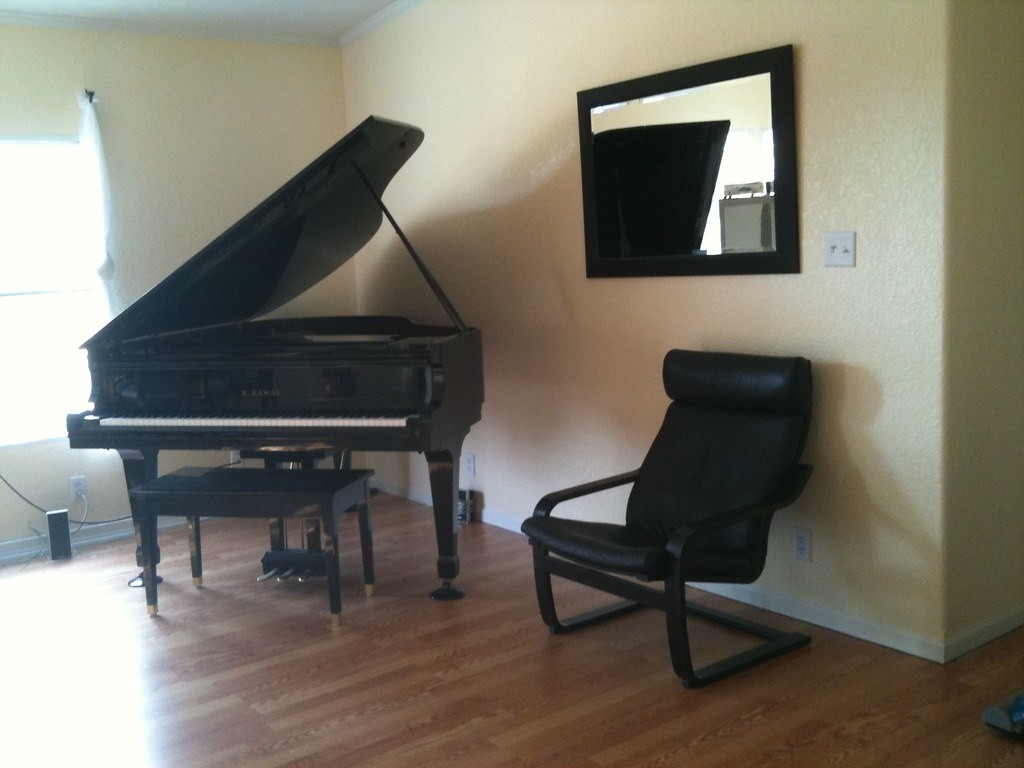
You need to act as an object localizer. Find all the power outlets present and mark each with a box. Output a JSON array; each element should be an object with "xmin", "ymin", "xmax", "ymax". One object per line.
[{"xmin": 792, "ymin": 526, "xmax": 812, "ymax": 564}]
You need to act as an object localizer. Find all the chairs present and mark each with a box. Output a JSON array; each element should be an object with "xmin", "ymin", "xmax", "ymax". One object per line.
[{"xmin": 521, "ymin": 350, "xmax": 815, "ymax": 689}]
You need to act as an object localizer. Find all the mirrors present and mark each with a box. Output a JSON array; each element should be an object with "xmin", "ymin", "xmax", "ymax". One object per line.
[{"xmin": 576, "ymin": 43, "xmax": 801, "ymax": 279}]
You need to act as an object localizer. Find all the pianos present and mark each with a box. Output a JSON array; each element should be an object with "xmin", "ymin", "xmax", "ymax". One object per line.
[{"xmin": 64, "ymin": 115, "xmax": 485, "ymax": 603}]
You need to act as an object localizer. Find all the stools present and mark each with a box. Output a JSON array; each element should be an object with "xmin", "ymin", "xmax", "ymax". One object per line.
[{"xmin": 129, "ymin": 465, "xmax": 375, "ymax": 629}]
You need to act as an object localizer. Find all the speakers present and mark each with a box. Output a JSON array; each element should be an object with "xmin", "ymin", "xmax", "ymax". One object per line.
[{"xmin": 46, "ymin": 509, "xmax": 71, "ymax": 560}]
